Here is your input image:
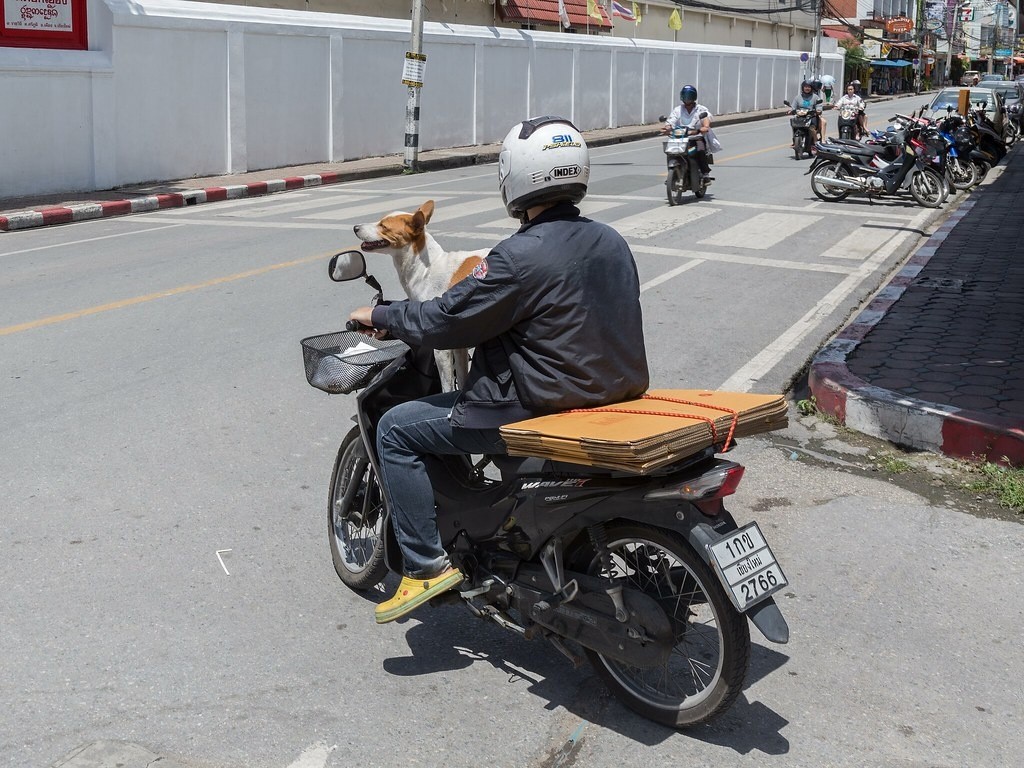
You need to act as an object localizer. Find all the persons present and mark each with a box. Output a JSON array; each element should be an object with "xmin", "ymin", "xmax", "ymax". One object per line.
[
  {"xmin": 823, "ymin": 86, "xmax": 835, "ymax": 102},
  {"xmin": 786, "ymin": 78, "xmax": 827, "ymax": 154},
  {"xmin": 834, "ymin": 80, "xmax": 871, "ymax": 140},
  {"xmin": 660, "ymin": 85, "xmax": 723, "ymax": 186},
  {"xmin": 973, "ymin": 74, "xmax": 979, "ymax": 86},
  {"xmin": 349, "ymin": 115, "xmax": 649, "ymax": 624}
]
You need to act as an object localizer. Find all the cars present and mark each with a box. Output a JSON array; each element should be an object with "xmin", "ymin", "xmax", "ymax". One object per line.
[{"xmin": 926, "ymin": 74, "xmax": 1024, "ymax": 136}]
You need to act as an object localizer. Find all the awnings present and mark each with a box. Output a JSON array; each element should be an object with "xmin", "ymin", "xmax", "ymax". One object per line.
[
  {"xmin": 822, "ymin": 28, "xmax": 861, "ymax": 48},
  {"xmin": 1009, "ymin": 57, "xmax": 1024, "ymax": 63},
  {"xmin": 870, "ymin": 59, "xmax": 913, "ymax": 66}
]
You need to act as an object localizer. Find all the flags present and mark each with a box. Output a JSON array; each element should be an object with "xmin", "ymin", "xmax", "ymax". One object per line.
[{"xmin": 558, "ymin": 0, "xmax": 682, "ymax": 30}]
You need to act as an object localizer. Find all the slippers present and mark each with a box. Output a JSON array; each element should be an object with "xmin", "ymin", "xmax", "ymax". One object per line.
[{"xmin": 375, "ymin": 566, "xmax": 463, "ymax": 624}]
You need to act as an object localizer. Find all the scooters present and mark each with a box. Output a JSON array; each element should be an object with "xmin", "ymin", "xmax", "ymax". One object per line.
[{"xmin": 837, "ymin": 106, "xmax": 863, "ymax": 142}]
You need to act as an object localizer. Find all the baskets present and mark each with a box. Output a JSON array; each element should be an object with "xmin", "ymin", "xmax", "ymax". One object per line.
[
  {"xmin": 791, "ymin": 118, "xmax": 809, "ymax": 127},
  {"xmin": 299, "ymin": 329, "xmax": 411, "ymax": 395},
  {"xmin": 663, "ymin": 142, "xmax": 688, "ymax": 157}
]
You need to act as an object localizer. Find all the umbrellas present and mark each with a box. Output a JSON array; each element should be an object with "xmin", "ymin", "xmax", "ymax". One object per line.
[{"xmin": 820, "ymin": 75, "xmax": 837, "ymax": 86}]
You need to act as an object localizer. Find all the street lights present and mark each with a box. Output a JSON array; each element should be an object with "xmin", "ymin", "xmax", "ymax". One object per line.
[{"xmin": 944, "ymin": 1, "xmax": 971, "ymax": 78}]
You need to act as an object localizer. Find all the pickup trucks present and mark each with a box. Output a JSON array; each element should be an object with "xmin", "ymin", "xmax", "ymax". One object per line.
[{"xmin": 961, "ymin": 70, "xmax": 982, "ymax": 85}]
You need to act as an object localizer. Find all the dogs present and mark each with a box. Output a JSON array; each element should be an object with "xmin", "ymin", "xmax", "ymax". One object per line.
[{"xmin": 352, "ymin": 199, "xmax": 494, "ymax": 395}]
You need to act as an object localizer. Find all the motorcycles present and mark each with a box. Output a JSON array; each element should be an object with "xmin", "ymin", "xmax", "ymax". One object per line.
[
  {"xmin": 300, "ymin": 251, "xmax": 790, "ymax": 727},
  {"xmin": 804, "ymin": 99, "xmax": 1021, "ymax": 208},
  {"xmin": 784, "ymin": 99, "xmax": 823, "ymax": 160},
  {"xmin": 658, "ymin": 111, "xmax": 715, "ymax": 206}
]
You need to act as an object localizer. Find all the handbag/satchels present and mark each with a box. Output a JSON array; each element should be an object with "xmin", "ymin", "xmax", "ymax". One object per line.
[{"xmin": 707, "ymin": 127, "xmax": 723, "ymax": 154}]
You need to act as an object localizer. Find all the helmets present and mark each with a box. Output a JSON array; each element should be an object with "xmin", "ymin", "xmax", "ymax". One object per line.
[
  {"xmin": 813, "ymin": 78, "xmax": 822, "ymax": 89},
  {"xmin": 802, "ymin": 80, "xmax": 813, "ymax": 87},
  {"xmin": 679, "ymin": 85, "xmax": 697, "ymax": 101},
  {"xmin": 498, "ymin": 115, "xmax": 590, "ymax": 219}
]
